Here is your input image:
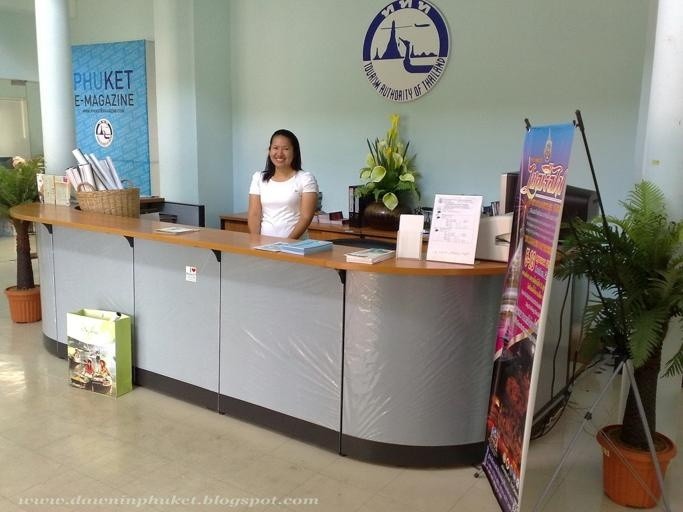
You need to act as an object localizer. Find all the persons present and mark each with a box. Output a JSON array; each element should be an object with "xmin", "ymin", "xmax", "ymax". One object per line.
[{"xmin": 247, "ymin": 129, "xmax": 319, "ymax": 240}]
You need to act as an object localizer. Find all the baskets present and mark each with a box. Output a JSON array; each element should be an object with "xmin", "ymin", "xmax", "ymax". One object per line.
[{"xmin": 76, "ymin": 178, "xmax": 141, "ymax": 218}]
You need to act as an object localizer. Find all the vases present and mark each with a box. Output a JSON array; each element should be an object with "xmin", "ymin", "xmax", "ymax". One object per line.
[{"xmin": 363, "ymin": 199, "xmax": 412, "ymax": 232}]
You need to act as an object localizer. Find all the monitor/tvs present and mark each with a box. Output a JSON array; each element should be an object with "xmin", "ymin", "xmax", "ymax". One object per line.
[
  {"xmin": 499, "ymin": 171, "xmax": 599, "ymax": 245},
  {"xmin": 160, "ymin": 202, "xmax": 205, "ymax": 227}
]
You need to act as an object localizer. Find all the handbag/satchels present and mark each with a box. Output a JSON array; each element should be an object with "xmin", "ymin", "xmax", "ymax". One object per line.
[{"xmin": 67, "ymin": 309, "xmax": 133, "ymax": 399}]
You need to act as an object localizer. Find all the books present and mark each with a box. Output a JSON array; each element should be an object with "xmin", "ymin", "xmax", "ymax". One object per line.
[
  {"xmin": 154, "ymin": 226, "xmax": 201, "ymax": 236},
  {"xmin": 253, "ymin": 239, "xmax": 395, "ymax": 265}
]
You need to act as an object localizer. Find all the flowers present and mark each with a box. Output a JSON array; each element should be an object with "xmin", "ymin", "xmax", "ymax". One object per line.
[{"xmin": 349, "ymin": 112, "xmax": 424, "ymax": 212}]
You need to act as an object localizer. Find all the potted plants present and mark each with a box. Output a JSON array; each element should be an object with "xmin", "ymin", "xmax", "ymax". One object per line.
[
  {"xmin": 546, "ymin": 176, "xmax": 683, "ymax": 512},
  {"xmin": 0, "ymin": 152, "xmax": 50, "ymax": 324}
]
